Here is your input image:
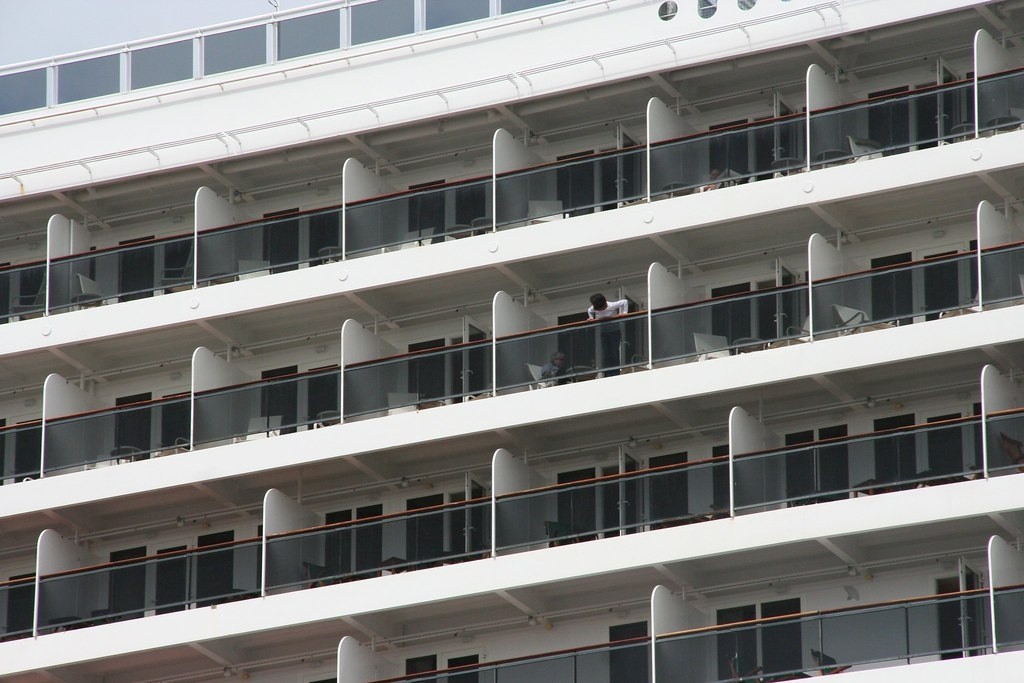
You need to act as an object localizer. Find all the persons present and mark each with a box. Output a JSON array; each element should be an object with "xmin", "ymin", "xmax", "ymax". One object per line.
[
  {"xmin": 541, "ymin": 351, "xmax": 574, "ymax": 385},
  {"xmin": 586, "ymin": 293, "xmax": 629, "ymax": 378},
  {"xmin": 700, "ymin": 168, "xmax": 726, "ymax": 194}
]
